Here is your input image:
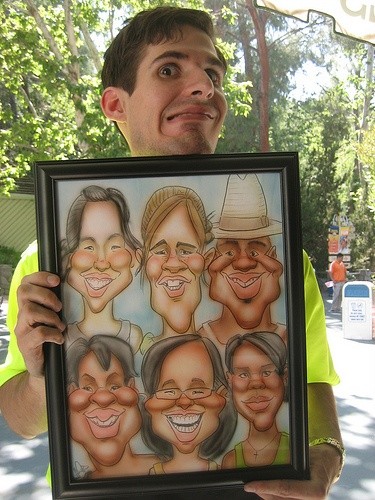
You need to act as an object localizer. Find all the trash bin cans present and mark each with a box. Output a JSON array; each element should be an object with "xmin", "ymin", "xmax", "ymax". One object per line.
[
  {"xmin": 341, "ymin": 280, "xmax": 375, "ymax": 340},
  {"xmin": 359, "ymin": 268, "xmax": 371, "ymax": 281}
]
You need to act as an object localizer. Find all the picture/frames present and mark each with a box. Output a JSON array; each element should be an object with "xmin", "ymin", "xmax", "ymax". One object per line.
[{"xmin": 32, "ymin": 150, "xmax": 314, "ymax": 500}]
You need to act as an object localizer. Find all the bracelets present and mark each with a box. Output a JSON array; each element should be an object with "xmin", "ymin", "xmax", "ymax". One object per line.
[{"xmin": 309, "ymin": 438, "xmax": 345, "ymax": 484}]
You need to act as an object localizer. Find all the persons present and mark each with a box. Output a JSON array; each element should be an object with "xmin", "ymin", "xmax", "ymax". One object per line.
[
  {"xmin": 328, "ymin": 253, "xmax": 349, "ymax": 311},
  {"xmin": 0, "ymin": 6, "xmax": 346, "ymax": 500}
]
